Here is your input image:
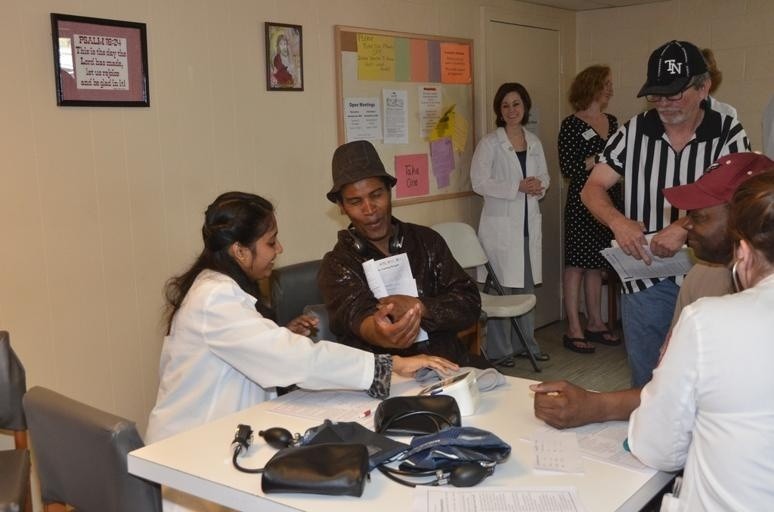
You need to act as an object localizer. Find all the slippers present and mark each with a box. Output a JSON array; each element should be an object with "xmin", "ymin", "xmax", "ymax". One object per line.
[
  {"xmin": 565, "ymin": 335, "xmax": 597, "ymax": 353},
  {"xmin": 585, "ymin": 328, "xmax": 623, "ymax": 346}
]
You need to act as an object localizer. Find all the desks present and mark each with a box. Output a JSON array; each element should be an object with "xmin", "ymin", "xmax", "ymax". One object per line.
[{"xmin": 128, "ymin": 359, "xmax": 682, "ymax": 512}]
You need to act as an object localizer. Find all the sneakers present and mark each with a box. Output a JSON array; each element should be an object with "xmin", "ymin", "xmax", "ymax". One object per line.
[
  {"xmin": 498, "ymin": 357, "xmax": 515, "ymax": 367},
  {"xmin": 519, "ymin": 350, "xmax": 550, "ymax": 361}
]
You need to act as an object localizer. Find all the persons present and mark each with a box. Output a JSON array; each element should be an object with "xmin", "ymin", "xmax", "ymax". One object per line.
[
  {"xmin": 272, "ymin": 35, "xmax": 297, "ymax": 87},
  {"xmin": 470, "ymin": 81, "xmax": 550, "ymax": 369},
  {"xmin": 627, "ymin": 172, "xmax": 773, "ymax": 511},
  {"xmin": 558, "ymin": 64, "xmax": 622, "ymax": 353},
  {"xmin": 528, "ymin": 153, "xmax": 774, "ymax": 429},
  {"xmin": 581, "ymin": 40, "xmax": 753, "ymax": 389},
  {"xmin": 143, "ymin": 191, "xmax": 460, "ymax": 446},
  {"xmin": 699, "ymin": 49, "xmax": 740, "ymax": 121},
  {"xmin": 317, "ymin": 140, "xmax": 481, "ymax": 367}
]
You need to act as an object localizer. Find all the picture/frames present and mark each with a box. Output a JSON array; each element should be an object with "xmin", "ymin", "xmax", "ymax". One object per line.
[
  {"xmin": 52, "ymin": 12, "xmax": 151, "ymax": 109},
  {"xmin": 266, "ymin": 20, "xmax": 303, "ymax": 92}
]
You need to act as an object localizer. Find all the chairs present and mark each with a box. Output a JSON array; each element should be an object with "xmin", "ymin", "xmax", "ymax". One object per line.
[
  {"xmin": 268, "ymin": 260, "xmax": 341, "ymax": 394},
  {"xmin": 430, "ymin": 222, "xmax": 544, "ymax": 375},
  {"xmin": 0, "ymin": 331, "xmax": 32, "ymax": 512},
  {"xmin": 23, "ymin": 387, "xmax": 161, "ymax": 512}
]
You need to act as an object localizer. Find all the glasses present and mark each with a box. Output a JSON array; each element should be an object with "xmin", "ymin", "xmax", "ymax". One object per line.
[{"xmin": 645, "ymin": 81, "xmax": 696, "ymax": 102}]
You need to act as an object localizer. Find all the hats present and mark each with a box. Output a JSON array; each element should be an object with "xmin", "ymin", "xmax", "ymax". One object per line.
[
  {"xmin": 662, "ymin": 152, "xmax": 774, "ymax": 210},
  {"xmin": 327, "ymin": 141, "xmax": 397, "ymax": 204},
  {"xmin": 636, "ymin": 41, "xmax": 709, "ymax": 99}
]
